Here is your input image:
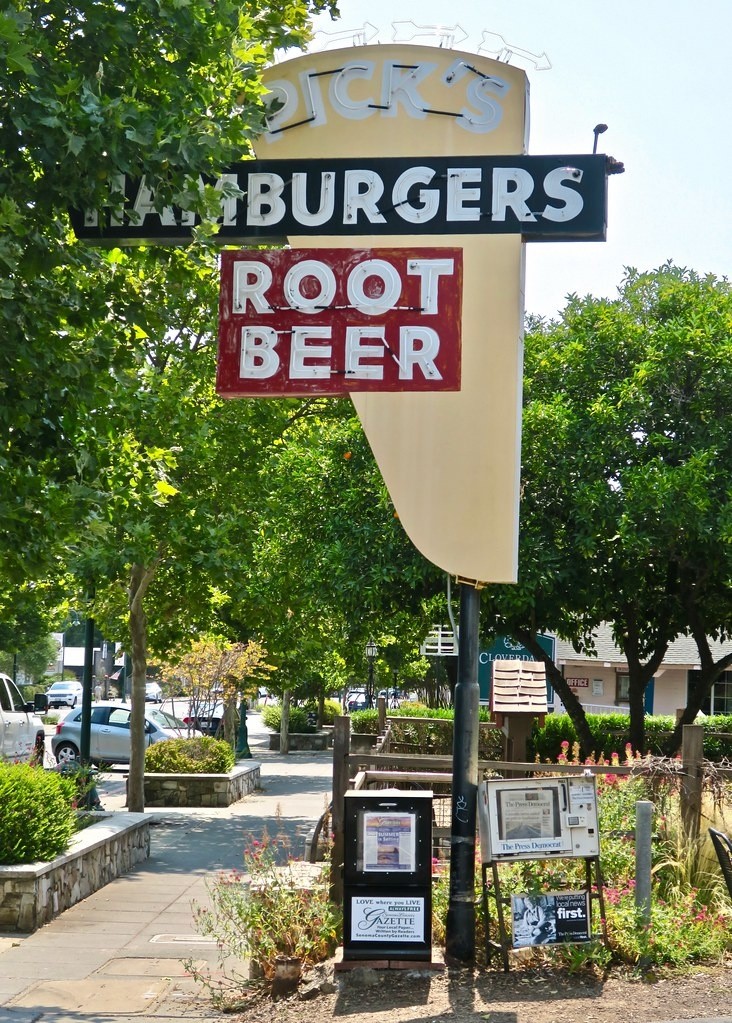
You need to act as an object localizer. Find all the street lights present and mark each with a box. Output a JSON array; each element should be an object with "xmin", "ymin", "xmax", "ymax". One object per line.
[
  {"xmin": 365, "ymin": 638, "xmax": 377, "ymax": 710},
  {"xmin": 393, "ymin": 665, "xmax": 399, "ymax": 700}
]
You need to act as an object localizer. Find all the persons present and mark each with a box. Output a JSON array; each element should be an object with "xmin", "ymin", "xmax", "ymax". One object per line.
[
  {"xmin": 523, "ymin": 896, "xmax": 548, "ymax": 942},
  {"xmin": 94, "ymin": 681, "xmax": 119, "ymax": 704}
]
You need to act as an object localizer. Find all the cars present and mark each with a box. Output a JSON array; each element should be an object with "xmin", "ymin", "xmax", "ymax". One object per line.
[
  {"xmin": 347, "ymin": 691, "xmax": 366, "ymax": 711},
  {"xmin": 182, "ymin": 702, "xmax": 226, "ymax": 739},
  {"xmin": 257, "ymin": 686, "xmax": 275, "ymax": 700},
  {"xmin": 44, "ymin": 681, "xmax": 84, "ymax": 709},
  {"xmin": 356, "ymin": 688, "xmax": 408, "ymax": 701},
  {"xmin": 145, "ymin": 683, "xmax": 163, "ymax": 704},
  {"xmin": 0, "ymin": 673, "xmax": 50, "ymax": 767},
  {"xmin": 51, "ymin": 702, "xmax": 211, "ymax": 766}
]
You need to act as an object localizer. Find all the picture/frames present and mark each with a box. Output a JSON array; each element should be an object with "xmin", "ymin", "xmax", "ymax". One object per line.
[{"xmin": 511, "ymin": 890, "xmax": 590, "ymax": 948}]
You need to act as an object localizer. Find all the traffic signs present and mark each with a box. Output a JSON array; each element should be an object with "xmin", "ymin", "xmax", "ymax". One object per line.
[
  {"xmin": 214, "ymin": 247, "xmax": 464, "ymax": 400},
  {"xmin": 64, "ymin": 152, "xmax": 625, "ymax": 243}
]
